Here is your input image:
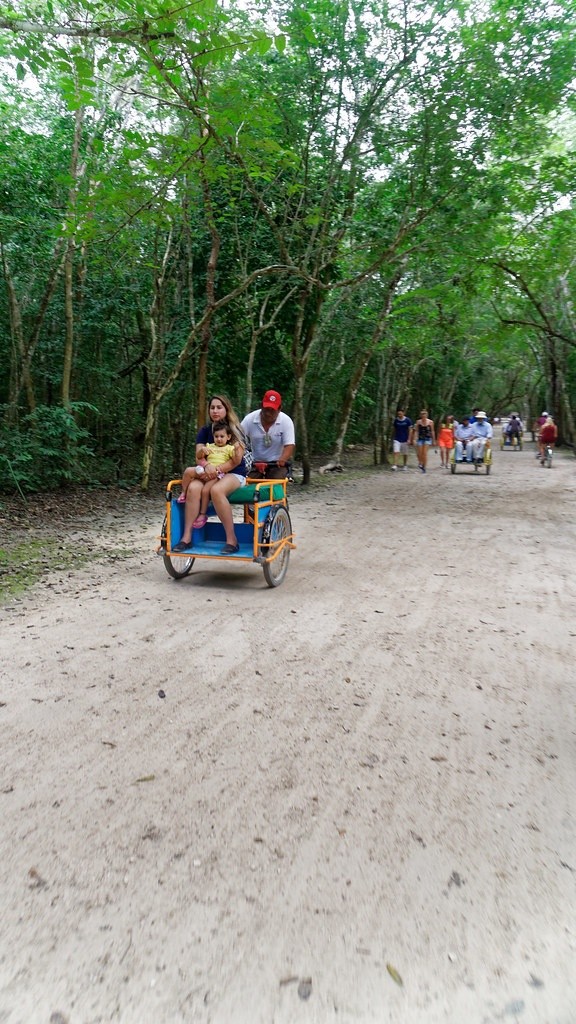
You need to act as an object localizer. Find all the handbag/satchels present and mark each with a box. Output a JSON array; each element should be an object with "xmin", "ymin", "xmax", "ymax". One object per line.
[{"xmin": 243, "ymin": 450, "xmax": 253, "ymax": 475}]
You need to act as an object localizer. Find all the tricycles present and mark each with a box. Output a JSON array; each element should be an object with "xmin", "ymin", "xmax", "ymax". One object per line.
[
  {"xmin": 450, "ymin": 440, "xmax": 492, "ymax": 475},
  {"xmin": 500, "ymin": 430, "xmax": 523, "ymax": 451},
  {"xmin": 157, "ymin": 462, "xmax": 297, "ymax": 588}
]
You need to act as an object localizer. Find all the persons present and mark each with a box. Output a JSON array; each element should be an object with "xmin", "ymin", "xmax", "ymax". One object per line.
[
  {"xmin": 502, "ymin": 416, "xmax": 523, "ymax": 445},
  {"xmin": 177, "ymin": 423, "xmax": 240, "ymax": 528},
  {"xmin": 533, "ymin": 412, "xmax": 557, "ymax": 465},
  {"xmin": 173, "ymin": 395, "xmax": 247, "ymax": 554},
  {"xmin": 436, "ymin": 409, "xmax": 500, "ymax": 465},
  {"xmin": 391, "ymin": 410, "xmax": 435, "ymax": 474},
  {"xmin": 240, "ymin": 391, "xmax": 296, "ymax": 480}
]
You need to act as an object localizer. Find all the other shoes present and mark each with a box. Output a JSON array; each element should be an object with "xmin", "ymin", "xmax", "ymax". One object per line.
[
  {"xmin": 467, "ymin": 460, "xmax": 472, "ymax": 464},
  {"xmin": 391, "ymin": 465, "xmax": 397, "ymax": 471},
  {"xmin": 172, "ymin": 542, "xmax": 192, "ymax": 551},
  {"xmin": 536, "ymin": 453, "xmax": 542, "ymax": 456},
  {"xmin": 402, "ymin": 466, "xmax": 408, "ymax": 470},
  {"xmin": 473, "ymin": 458, "xmax": 482, "ymax": 463},
  {"xmin": 446, "ymin": 464, "xmax": 451, "ymax": 469},
  {"xmin": 456, "ymin": 460, "xmax": 461, "ymax": 463},
  {"xmin": 440, "ymin": 464, "xmax": 445, "ymax": 468},
  {"xmin": 418, "ymin": 464, "xmax": 425, "ymax": 473},
  {"xmin": 177, "ymin": 494, "xmax": 186, "ymax": 503},
  {"xmin": 193, "ymin": 516, "xmax": 208, "ymax": 528},
  {"xmin": 540, "ymin": 460, "xmax": 545, "ymax": 464},
  {"xmin": 221, "ymin": 543, "xmax": 239, "ymax": 554}
]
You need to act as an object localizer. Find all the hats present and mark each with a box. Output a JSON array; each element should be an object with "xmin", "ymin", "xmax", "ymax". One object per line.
[
  {"xmin": 475, "ymin": 411, "xmax": 487, "ymax": 418},
  {"xmin": 263, "ymin": 390, "xmax": 281, "ymax": 410},
  {"xmin": 512, "ymin": 416, "xmax": 516, "ymax": 419},
  {"xmin": 542, "ymin": 412, "xmax": 548, "ymax": 416}
]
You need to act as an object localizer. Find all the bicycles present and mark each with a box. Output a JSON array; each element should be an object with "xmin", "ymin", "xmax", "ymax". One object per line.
[{"xmin": 535, "ymin": 436, "xmax": 553, "ymax": 468}]
